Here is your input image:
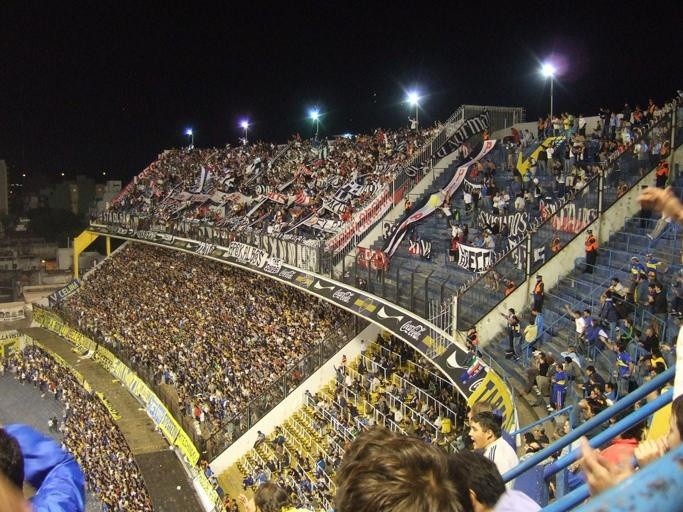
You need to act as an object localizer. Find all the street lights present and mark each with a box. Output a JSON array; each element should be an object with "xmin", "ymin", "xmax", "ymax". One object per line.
[
  {"xmin": 312, "ymin": 103, "xmax": 320, "ymax": 141},
  {"xmin": 541, "ymin": 59, "xmax": 553, "ymax": 125},
  {"xmin": 242, "ymin": 117, "xmax": 247, "ymax": 142},
  {"xmin": 409, "ymin": 90, "xmax": 418, "ymax": 133},
  {"xmin": 187, "ymin": 130, "xmax": 194, "ymax": 147}
]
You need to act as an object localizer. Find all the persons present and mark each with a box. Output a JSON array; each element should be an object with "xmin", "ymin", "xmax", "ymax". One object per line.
[{"xmin": 1, "ymin": 97, "xmax": 683, "ymax": 512}]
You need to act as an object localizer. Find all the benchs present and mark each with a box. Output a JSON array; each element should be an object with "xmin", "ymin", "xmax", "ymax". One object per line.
[{"xmin": 235, "ymin": 127, "xmax": 682, "ymax": 512}]
